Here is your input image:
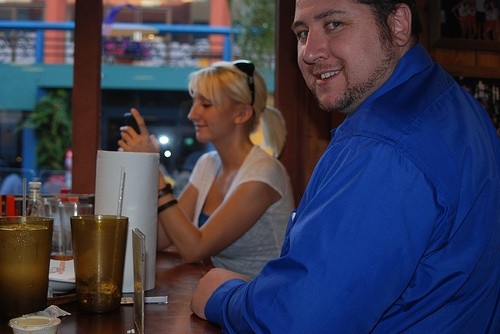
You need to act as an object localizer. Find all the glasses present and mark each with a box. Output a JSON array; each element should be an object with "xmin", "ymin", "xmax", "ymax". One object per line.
[{"xmin": 233, "ymin": 60, "xmax": 255, "ymax": 107}]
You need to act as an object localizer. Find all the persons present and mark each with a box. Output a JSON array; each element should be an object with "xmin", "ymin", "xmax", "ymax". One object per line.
[
  {"xmin": 0, "ymin": 168, "xmax": 67, "ymax": 198},
  {"xmin": 191, "ymin": 0, "xmax": 500, "ymax": 334},
  {"xmin": 119, "ymin": 60, "xmax": 296, "ymax": 282}
]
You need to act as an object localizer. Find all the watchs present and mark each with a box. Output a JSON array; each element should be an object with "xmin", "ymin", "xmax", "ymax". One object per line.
[{"xmin": 158, "ymin": 182, "xmax": 174, "ymax": 198}]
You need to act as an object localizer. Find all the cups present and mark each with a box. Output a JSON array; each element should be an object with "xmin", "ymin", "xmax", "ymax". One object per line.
[
  {"xmin": 42, "ymin": 196, "xmax": 93, "ymax": 263},
  {"xmin": 0, "ymin": 216, "xmax": 54, "ymax": 324},
  {"xmin": 69, "ymin": 213, "xmax": 128, "ymax": 314}
]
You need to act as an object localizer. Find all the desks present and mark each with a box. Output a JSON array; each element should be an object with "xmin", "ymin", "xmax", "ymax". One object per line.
[{"xmin": 0, "ymin": 251, "xmax": 223, "ymax": 334}]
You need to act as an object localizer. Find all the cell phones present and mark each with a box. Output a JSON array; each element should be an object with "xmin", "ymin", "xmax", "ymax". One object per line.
[{"xmin": 122, "ymin": 111, "xmax": 141, "ymax": 135}]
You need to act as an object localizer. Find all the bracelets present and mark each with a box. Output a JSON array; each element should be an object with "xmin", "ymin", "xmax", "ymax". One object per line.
[{"xmin": 157, "ymin": 199, "xmax": 177, "ymax": 215}]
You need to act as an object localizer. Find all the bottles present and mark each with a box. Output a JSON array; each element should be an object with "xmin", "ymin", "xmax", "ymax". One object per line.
[{"xmin": 25, "ymin": 182, "xmax": 46, "ymax": 217}]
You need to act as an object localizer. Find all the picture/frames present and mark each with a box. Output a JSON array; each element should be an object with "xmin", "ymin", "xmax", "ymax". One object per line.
[{"xmin": 426, "ymin": 0, "xmax": 500, "ymax": 53}]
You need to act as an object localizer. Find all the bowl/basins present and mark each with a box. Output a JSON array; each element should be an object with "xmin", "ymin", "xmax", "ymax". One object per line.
[{"xmin": 8, "ymin": 316, "xmax": 61, "ymax": 334}]
